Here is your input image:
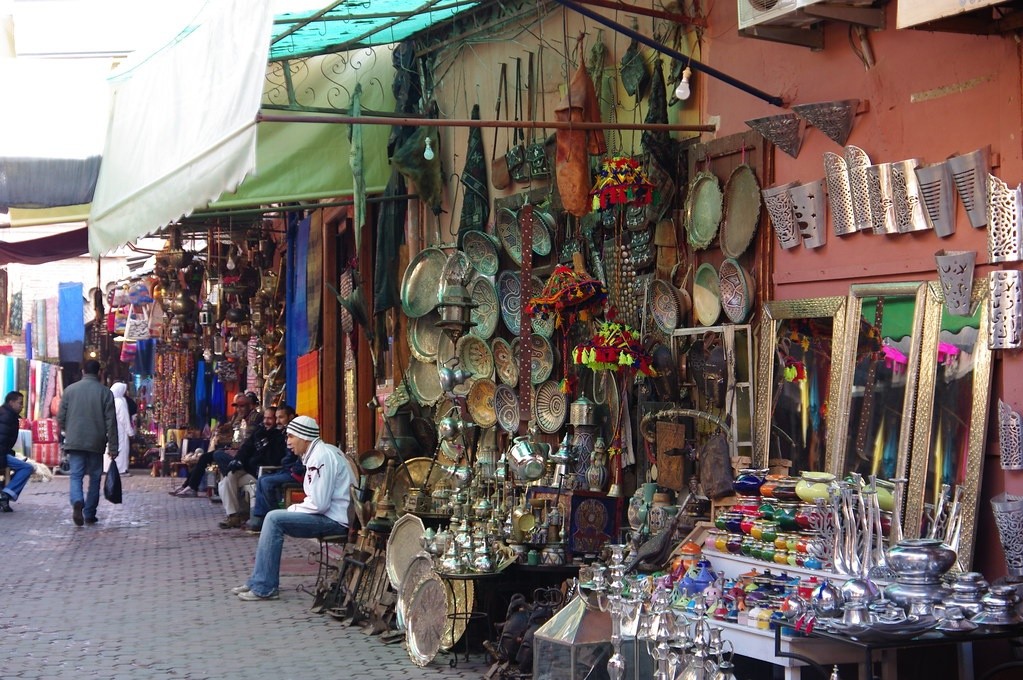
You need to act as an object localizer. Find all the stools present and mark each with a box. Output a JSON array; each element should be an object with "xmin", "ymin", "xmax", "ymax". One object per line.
[
  {"xmin": 244, "ymin": 462, "xmax": 282, "ymax": 522},
  {"xmin": 295, "ymin": 534, "xmax": 348, "ymax": 600},
  {"xmin": 285, "ymin": 486, "xmax": 305, "ymax": 510}
]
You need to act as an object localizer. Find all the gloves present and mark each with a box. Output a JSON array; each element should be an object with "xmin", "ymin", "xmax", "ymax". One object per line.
[{"xmin": 223, "ymin": 459, "xmax": 243, "ymax": 477}]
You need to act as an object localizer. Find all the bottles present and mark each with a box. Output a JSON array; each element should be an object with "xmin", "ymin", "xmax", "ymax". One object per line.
[{"xmin": 706, "ymin": 465, "xmax": 957, "ymax": 630}]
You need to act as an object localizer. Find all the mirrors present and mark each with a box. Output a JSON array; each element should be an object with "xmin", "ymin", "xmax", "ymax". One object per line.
[
  {"xmin": 756, "ymin": 295, "xmax": 845, "ymax": 473},
  {"xmin": 906, "ymin": 279, "xmax": 993, "ymax": 579},
  {"xmin": 836, "ymin": 281, "xmax": 926, "ymax": 528}
]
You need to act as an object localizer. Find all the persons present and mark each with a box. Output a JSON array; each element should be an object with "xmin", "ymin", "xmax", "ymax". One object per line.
[
  {"xmin": 231, "ymin": 415, "xmax": 361, "ymax": 601},
  {"xmin": 218, "ymin": 407, "xmax": 306, "ymax": 533},
  {"xmin": 0, "ymin": 391, "xmax": 34, "ymax": 513},
  {"xmin": 102, "ymin": 383, "xmax": 135, "ymax": 477},
  {"xmin": 56, "ymin": 359, "xmax": 119, "ymax": 526},
  {"xmin": 113, "ymin": 380, "xmax": 136, "ymax": 415},
  {"xmin": 169, "ymin": 390, "xmax": 263, "ymax": 501}
]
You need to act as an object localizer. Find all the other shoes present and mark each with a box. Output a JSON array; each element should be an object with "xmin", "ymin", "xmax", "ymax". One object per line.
[
  {"xmin": 73, "ymin": 503, "xmax": 83, "ymax": 526},
  {"xmin": 246, "ymin": 515, "xmax": 263, "ymax": 531},
  {"xmin": 0, "ymin": 491, "xmax": 13, "ymax": 512},
  {"xmin": 84, "ymin": 516, "xmax": 99, "ymax": 523}
]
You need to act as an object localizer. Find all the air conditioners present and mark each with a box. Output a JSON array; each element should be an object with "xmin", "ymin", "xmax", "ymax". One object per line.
[{"xmin": 737, "ymin": 0, "xmax": 872, "ymax": 31}]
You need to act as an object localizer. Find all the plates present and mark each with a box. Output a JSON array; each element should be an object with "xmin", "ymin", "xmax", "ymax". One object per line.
[{"xmin": 383, "ymin": 156, "xmax": 760, "ymax": 669}]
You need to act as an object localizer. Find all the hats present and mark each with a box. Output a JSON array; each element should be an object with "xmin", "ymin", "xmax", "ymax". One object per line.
[
  {"xmin": 286, "ymin": 415, "xmax": 320, "ymax": 442},
  {"xmin": 231, "ymin": 396, "xmax": 251, "ymax": 406}
]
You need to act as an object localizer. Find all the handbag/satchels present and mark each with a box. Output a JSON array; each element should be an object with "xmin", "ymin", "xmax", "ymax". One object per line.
[{"xmin": 104, "ymin": 458, "xmax": 122, "ymax": 504}]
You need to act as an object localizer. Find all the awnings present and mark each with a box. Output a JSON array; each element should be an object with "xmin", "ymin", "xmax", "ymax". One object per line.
[{"xmin": 0, "ymin": 0, "xmax": 779, "ymax": 267}]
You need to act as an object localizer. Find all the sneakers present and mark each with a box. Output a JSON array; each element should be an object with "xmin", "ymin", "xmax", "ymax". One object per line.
[
  {"xmin": 170, "ymin": 486, "xmax": 184, "ymax": 497},
  {"xmin": 238, "ymin": 588, "xmax": 279, "ymax": 599},
  {"xmin": 231, "ymin": 585, "xmax": 249, "ymax": 595},
  {"xmin": 218, "ymin": 513, "xmax": 241, "ymax": 528},
  {"xmin": 177, "ymin": 485, "xmax": 199, "ymax": 497}
]
